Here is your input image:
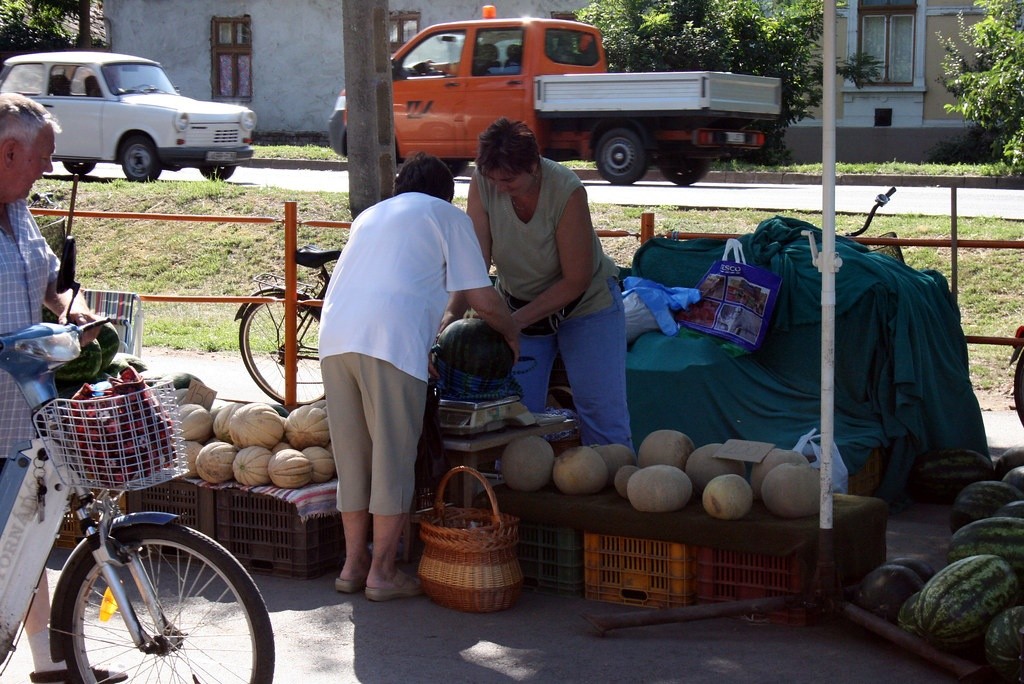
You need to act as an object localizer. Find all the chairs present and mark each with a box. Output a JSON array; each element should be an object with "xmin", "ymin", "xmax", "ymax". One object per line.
[
  {"xmin": 554, "ymin": 37, "xmax": 578, "ymax": 64},
  {"xmin": 504, "ymin": 44, "xmax": 522, "ymax": 75},
  {"xmin": 472, "ymin": 44, "xmax": 501, "ymax": 76},
  {"xmin": 81, "ymin": 290, "xmax": 142, "ymax": 358},
  {"xmin": 84, "ymin": 74, "xmax": 103, "ymax": 98},
  {"xmin": 50, "ymin": 75, "xmax": 72, "ymax": 96}
]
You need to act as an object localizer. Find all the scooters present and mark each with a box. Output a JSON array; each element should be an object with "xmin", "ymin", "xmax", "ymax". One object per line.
[{"xmin": 1, "ymin": 235, "xmax": 275, "ymax": 684}]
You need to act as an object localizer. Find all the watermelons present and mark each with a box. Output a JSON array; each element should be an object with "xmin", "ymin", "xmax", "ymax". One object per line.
[
  {"xmin": 853, "ymin": 448, "xmax": 1024, "ymax": 684},
  {"xmin": 435, "ymin": 317, "xmax": 515, "ymax": 380},
  {"xmin": 52, "ymin": 319, "xmax": 134, "ymax": 396}
]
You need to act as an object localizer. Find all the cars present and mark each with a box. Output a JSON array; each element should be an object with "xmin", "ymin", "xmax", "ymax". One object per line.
[{"xmin": 0, "ymin": 52, "xmax": 259, "ymax": 185}]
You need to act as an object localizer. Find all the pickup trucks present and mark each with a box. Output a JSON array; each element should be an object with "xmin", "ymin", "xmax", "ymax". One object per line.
[{"xmin": 327, "ymin": 4, "xmax": 783, "ymax": 187}]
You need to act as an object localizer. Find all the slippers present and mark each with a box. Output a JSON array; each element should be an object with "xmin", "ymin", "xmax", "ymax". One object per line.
[
  {"xmin": 365, "ymin": 568, "xmax": 421, "ymax": 602},
  {"xmin": 335, "ymin": 575, "xmax": 367, "ymax": 593}
]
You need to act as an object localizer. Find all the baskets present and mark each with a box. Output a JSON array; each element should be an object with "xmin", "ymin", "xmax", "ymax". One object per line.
[
  {"xmin": 31, "ymin": 378, "xmax": 188, "ymax": 493},
  {"xmin": 867, "ymin": 230, "xmax": 906, "ymax": 264},
  {"xmin": 417, "ymin": 466, "xmax": 523, "ymax": 612}
]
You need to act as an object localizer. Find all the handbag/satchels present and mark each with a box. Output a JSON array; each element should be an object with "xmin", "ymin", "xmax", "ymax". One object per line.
[
  {"xmin": 499, "ymin": 285, "xmax": 585, "ymax": 338},
  {"xmin": 434, "ymin": 356, "xmax": 538, "ymax": 403},
  {"xmin": 674, "ymin": 238, "xmax": 782, "ymax": 350},
  {"xmin": 792, "ymin": 428, "xmax": 850, "ymax": 495}
]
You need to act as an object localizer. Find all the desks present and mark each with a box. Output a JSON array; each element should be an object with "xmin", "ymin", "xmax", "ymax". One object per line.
[{"xmin": 442, "ymin": 413, "xmax": 578, "ymax": 507}]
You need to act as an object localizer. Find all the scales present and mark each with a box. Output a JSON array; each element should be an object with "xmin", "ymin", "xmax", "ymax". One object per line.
[{"xmin": 438, "ymin": 393, "xmax": 536, "ymax": 440}]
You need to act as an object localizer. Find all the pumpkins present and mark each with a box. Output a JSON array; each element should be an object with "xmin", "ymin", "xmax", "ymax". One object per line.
[
  {"xmin": 168, "ymin": 399, "xmax": 337, "ymax": 489},
  {"xmin": 501, "ymin": 428, "xmax": 821, "ymax": 519}
]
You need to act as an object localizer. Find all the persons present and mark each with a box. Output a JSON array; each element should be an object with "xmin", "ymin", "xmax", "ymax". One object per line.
[
  {"xmin": 316, "ymin": 148, "xmax": 513, "ymax": 601},
  {"xmin": 0, "ymin": 91, "xmax": 130, "ymax": 684},
  {"xmin": 438, "ymin": 119, "xmax": 639, "ymax": 467}
]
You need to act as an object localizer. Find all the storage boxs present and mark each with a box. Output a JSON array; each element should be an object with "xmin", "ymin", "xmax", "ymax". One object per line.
[
  {"xmin": 57, "ymin": 467, "xmax": 346, "ymax": 580},
  {"xmin": 484, "ymin": 489, "xmax": 887, "ymax": 614}
]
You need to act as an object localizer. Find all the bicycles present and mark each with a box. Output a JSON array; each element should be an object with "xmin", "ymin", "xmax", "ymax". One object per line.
[{"xmin": 233, "ymin": 244, "xmax": 342, "ymax": 410}]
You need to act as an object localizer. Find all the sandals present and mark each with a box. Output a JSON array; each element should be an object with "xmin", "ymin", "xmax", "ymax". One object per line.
[{"xmin": 30, "ymin": 665, "xmax": 128, "ymax": 684}]
